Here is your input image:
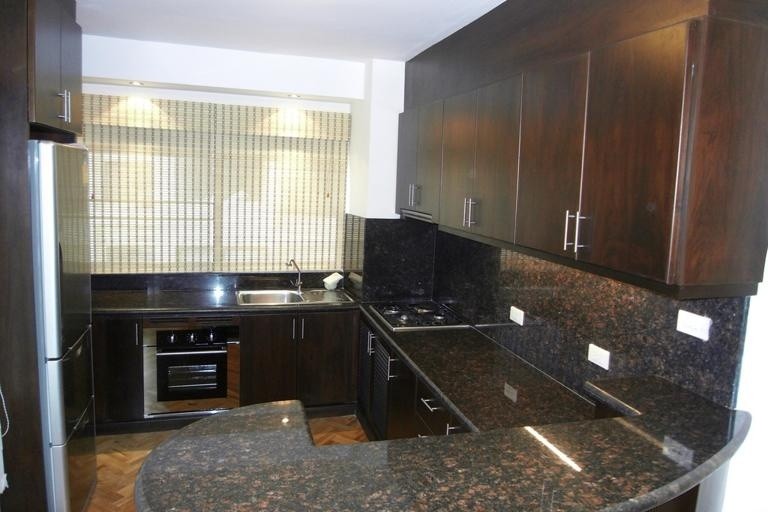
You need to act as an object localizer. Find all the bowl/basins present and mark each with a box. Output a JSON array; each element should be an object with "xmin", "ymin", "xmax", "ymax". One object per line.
[{"xmin": 323, "ymin": 281, "xmax": 339, "ymax": 291}]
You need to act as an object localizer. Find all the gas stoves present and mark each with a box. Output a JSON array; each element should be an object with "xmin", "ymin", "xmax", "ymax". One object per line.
[{"xmin": 368, "ymin": 296, "xmax": 471, "ymax": 333}]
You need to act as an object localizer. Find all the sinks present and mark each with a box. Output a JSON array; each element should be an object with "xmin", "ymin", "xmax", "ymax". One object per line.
[{"xmin": 235, "ymin": 289, "xmax": 304, "ymax": 306}]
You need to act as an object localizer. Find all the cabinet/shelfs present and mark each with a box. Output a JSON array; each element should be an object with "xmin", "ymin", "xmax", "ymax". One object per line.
[
  {"xmin": 358, "ymin": 312, "xmax": 470, "ymax": 442},
  {"xmin": 239, "ymin": 308, "xmax": 359, "ymax": 410},
  {"xmin": 27, "ymin": 0, "xmax": 84, "ymax": 139},
  {"xmin": 343, "ymin": 213, "xmax": 436, "ymax": 305},
  {"xmin": 395, "ymin": 0, "xmax": 768, "ymax": 298},
  {"xmin": 90, "ymin": 314, "xmax": 144, "ymax": 419}
]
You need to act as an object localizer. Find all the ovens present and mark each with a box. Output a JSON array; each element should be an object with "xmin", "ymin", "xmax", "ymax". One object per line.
[{"xmin": 142, "ymin": 325, "xmax": 242, "ymax": 418}]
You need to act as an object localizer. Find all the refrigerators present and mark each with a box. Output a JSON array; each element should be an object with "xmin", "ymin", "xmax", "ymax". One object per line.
[{"xmin": 25, "ymin": 139, "xmax": 98, "ymax": 512}]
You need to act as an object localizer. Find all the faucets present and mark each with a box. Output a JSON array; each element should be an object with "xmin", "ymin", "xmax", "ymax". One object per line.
[{"xmin": 285, "ymin": 259, "xmax": 303, "ymax": 291}]
